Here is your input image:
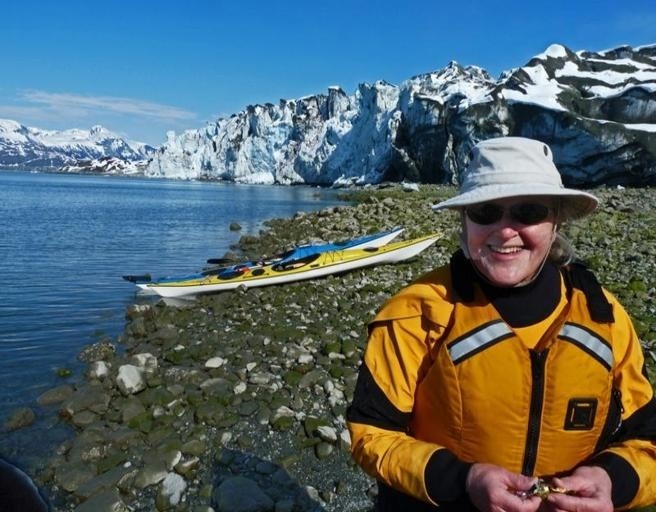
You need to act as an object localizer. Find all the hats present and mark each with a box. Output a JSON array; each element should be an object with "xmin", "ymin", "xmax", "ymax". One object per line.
[{"xmin": 431, "ymin": 132, "xmax": 602, "ymax": 222}]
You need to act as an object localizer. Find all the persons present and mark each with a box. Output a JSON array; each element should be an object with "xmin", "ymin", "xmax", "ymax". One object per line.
[{"xmin": 341, "ymin": 136, "xmax": 655, "ymax": 512}]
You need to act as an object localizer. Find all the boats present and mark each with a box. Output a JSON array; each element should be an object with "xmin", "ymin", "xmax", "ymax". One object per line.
[
  {"xmin": 137, "ymin": 232, "xmax": 445, "ymax": 300},
  {"xmin": 122, "ymin": 227, "xmax": 406, "ymax": 297}
]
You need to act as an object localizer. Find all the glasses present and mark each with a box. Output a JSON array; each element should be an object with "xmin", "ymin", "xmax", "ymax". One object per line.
[{"xmin": 461, "ymin": 200, "xmax": 561, "ymax": 227}]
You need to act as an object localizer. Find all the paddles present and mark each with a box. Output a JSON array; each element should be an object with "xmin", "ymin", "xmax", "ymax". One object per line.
[
  {"xmin": 217, "ymin": 261, "xmax": 280, "ymax": 281},
  {"xmin": 207, "ymin": 251, "xmax": 296, "ymax": 264},
  {"xmin": 201, "ymin": 265, "xmax": 245, "ymax": 275},
  {"xmin": 123, "ymin": 274, "xmax": 152, "ymax": 283}
]
